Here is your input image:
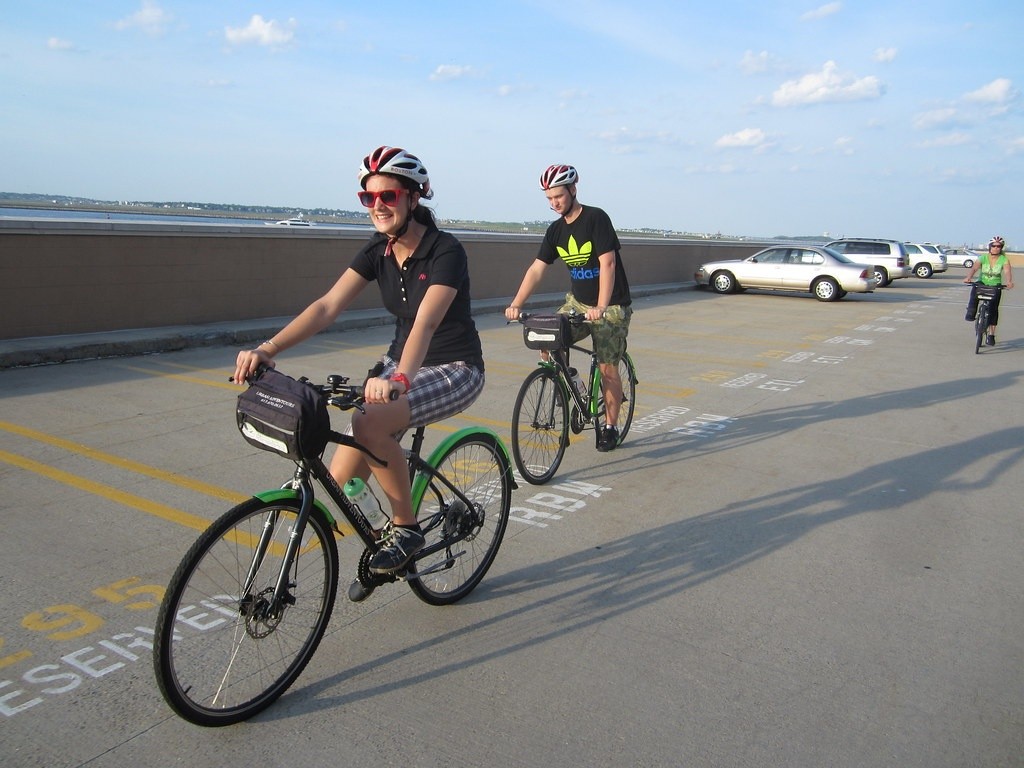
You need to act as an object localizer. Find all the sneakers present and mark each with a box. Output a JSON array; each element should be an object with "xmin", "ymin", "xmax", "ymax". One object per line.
[
  {"xmin": 556, "ymin": 394, "xmax": 562, "ymax": 407},
  {"xmin": 597, "ymin": 429, "xmax": 619, "ymax": 452},
  {"xmin": 368, "ymin": 527, "xmax": 426, "ymax": 573},
  {"xmin": 348, "ymin": 576, "xmax": 375, "ymax": 603},
  {"xmin": 988, "ymin": 335, "xmax": 995, "ymax": 345},
  {"xmin": 965, "ymin": 316, "xmax": 975, "ymax": 321}
]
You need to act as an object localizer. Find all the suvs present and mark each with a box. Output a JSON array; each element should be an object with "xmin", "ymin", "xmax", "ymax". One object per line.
[
  {"xmin": 903, "ymin": 243, "xmax": 948, "ymax": 278},
  {"xmin": 791, "ymin": 237, "xmax": 912, "ymax": 288}
]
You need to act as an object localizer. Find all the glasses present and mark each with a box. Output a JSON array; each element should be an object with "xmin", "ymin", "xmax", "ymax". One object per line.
[
  {"xmin": 991, "ymin": 244, "xmax": 1002, "ymax": 248},
  {"xmin": 359, "ymin": 189, "xmax": 409, "ymax": 208}
]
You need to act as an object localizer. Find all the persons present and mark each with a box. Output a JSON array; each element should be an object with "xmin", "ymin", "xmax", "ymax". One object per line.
[
  {"xmin": 965, "ymin": 237, "xmax": 1013, "ymax": 346},
  {"xmin": 504, "ymin": 165, "xmax": 633, "ymax": 452},
  {"xmin": 232, "ymin": 146, "xmax": 486, "ymax": 603}
]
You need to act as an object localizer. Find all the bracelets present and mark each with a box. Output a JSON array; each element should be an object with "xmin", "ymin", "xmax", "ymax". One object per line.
[{"xmin": 264, "ymin": 340, "xmax": 280, "ymax": 353}]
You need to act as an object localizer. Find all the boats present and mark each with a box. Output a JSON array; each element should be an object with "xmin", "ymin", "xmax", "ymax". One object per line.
[{"xmin": 263, "ymin": 215, "xmax": 317, "ymax": 226}]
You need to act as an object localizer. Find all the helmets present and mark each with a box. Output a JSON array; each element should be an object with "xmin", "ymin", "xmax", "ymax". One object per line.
[
  {"xmin": 539, "ymin": 164, "xmax": 577, "ymax": 190},
  {"xmin": 989, "ymin": 236, "xmax": 1005, "ymax": 246},
  {"xmin": 358, "ymin": 146, "xmax": 430, "ymax": 196}
]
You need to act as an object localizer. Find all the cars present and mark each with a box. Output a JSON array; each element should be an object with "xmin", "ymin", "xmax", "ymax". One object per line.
[
  {"xmin": 694, "ymin": 244, "xmax": 877, "ymax": 303},
  {"xmin": 944, "ymin": 249, "xmax": 981, "ymax": 268}
]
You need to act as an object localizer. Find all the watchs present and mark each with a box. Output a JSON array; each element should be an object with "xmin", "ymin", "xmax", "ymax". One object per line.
[{"xmin": 389, "ymin": 372, "xmax": 410, "ymax": 394}]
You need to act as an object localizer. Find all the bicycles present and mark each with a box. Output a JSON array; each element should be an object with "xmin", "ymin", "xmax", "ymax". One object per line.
[
  {"xmin": 965, "ymin": 282, "xmax": 1009, "ymax": 354},
  {"xmin": 153, "ymin": 364, "xmax": 520, "ymax": 728},
  {"xmin": 505, "ymin": 313, "xmax": 640, "ymax": 485}
]
